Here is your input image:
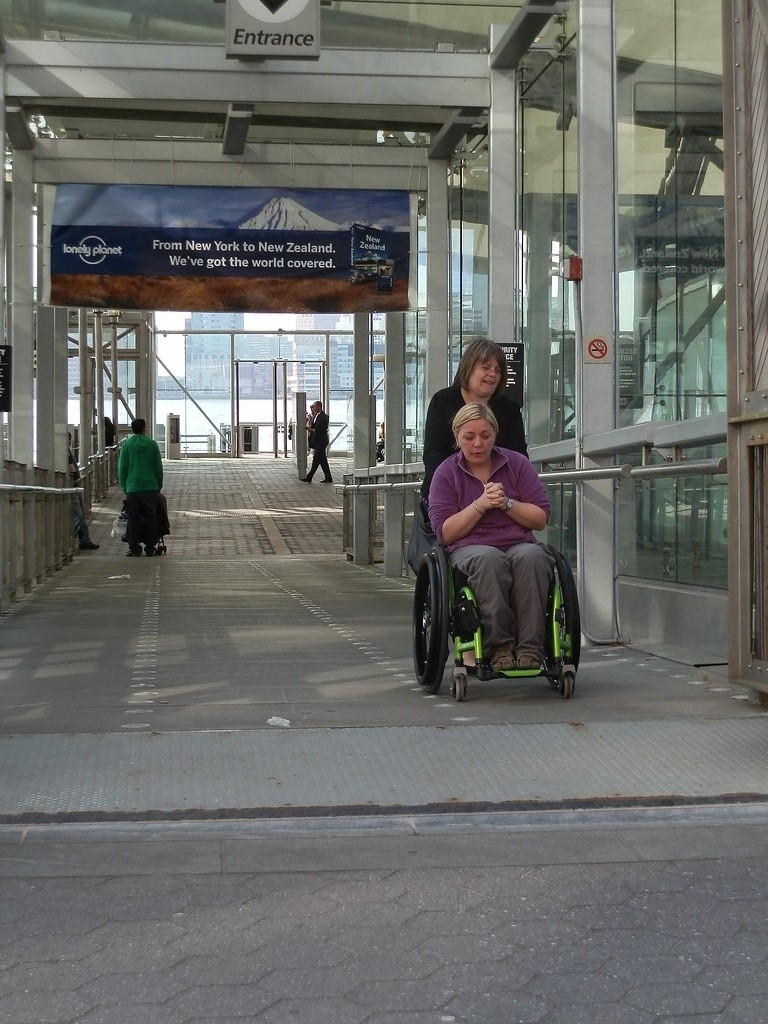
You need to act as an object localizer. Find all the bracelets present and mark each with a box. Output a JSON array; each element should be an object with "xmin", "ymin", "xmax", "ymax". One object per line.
[{"xmin": 474, "ymin": 500, "xmax": 484, "ymax": 514}]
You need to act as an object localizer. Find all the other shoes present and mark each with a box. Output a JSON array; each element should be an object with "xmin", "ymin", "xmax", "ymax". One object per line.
[
  {"xmin": 490, "ymin": 649, "xmax": 516, "ymax": 672},
  {"xmin": 516, "ymin": 652, "xmax": 540, "ymax": 669},
  {"xmin": 79, "ymin": 542, "xmax": 99, "ymax": 550},
  {"xmin": 300, "ymin": 477, "xmax": 312, "ymax": 483},
  {"xmin": 146, "ymin": 550, "xmax": 154, "ymax": 556},
  {"xmin": 126, "ymin": 551, "xmax": 141, "ymax": 557},
  {"xmin": 320, "ymin": 477, "xmax": 332, "ymax": 484},
  {"xmin": 463, "ymin": 664, "xmax": 477, "ymax": 677}
]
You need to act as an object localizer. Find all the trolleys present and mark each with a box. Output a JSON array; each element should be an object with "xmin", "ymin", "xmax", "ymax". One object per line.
[{"xmin": 120, "ymin": 494, "xmax": 171, "ymax": 556}]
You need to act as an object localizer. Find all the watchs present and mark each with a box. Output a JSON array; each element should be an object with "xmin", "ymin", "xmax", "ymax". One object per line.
[{"xmin": 503, "ymin": 498, "xmax": 513, "ymax": 512}]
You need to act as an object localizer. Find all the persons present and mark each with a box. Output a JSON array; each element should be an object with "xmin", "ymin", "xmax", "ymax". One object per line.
[
  {"xmin": 68, "ymin": 432, "xmax": 100, "ymax": 550},
  {"xmin": 118, "ymin": 419, "xmax": 163, "ymax": 556},
  {"xmin": 105, "ymin": 417, "xmax": 115, "ymax": 447},
  {"xmin": 376, "ymin": 422, "xmax": 385, "ymax": 462},
  {"xmin": 300, "ymin": 400, "xmax": 333, "ymax": 483},
  {"xmin": 429, "ymin": 402, "xmax": 550, "ymax": 671},
  {"xmin": 423, "ymin": 340, "xmax": 530, "ymax": 676}
]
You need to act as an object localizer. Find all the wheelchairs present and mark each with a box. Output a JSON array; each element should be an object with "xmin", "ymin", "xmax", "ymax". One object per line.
[{"xmin": 410, "ymin": 544, "xmax": 582, "ymax": 703}]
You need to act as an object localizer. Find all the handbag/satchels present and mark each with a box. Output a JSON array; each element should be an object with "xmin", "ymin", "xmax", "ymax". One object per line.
[
  {"xmin": 111, "ymin": 518, "xmax": 128, "ymax": 539},
  {"xmin": 407, "ymin": 504, "xmax": 440, "ymax": 577}
]
